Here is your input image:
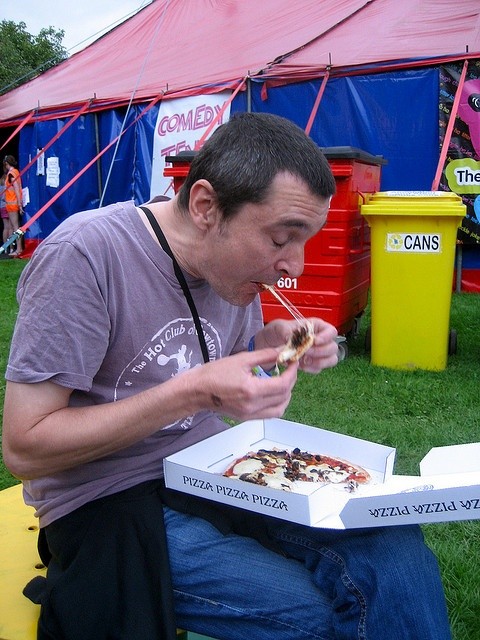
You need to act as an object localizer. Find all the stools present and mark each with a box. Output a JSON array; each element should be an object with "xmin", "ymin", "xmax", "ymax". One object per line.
[{"xmin": 0, "ymin": 481, "xmax": 187, "ymax": 639}]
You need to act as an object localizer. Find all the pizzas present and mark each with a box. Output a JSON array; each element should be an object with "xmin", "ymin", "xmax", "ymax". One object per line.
[
  {"xmin": 220, "ymin": 447, "xmax": 371, "ymax": 493},
  {"xmin": 276, "ymin": 322, "xmax": 316, "ymax": 363}
]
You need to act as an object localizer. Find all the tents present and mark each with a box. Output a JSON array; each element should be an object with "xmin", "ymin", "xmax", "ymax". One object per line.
[{"xmin": 1, "ymin": 0, "xmax": 478, "ymax": 271}]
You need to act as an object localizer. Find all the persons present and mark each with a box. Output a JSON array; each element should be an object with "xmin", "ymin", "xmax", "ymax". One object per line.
[
  {"xmin": 2, "ymin": 110, "xmax": 459, "ymax": 640},
  {"xmin": 0, "ymin": 175, "xmax": 14, "ymax": 255},
  {"xmin": 5, "ymin": 155, "xmax": 25, "ymax": 257}
]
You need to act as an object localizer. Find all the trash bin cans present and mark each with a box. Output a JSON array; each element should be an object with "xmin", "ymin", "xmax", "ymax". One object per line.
[{"xmin": 360, "ymin": 192, "xmax": 468, "ymax": 372}]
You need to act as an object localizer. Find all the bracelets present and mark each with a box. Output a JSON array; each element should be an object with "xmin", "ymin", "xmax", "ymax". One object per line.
[{"xmin": 18, "ymin": 204, "xmax": 22, "ymax": 207}]
